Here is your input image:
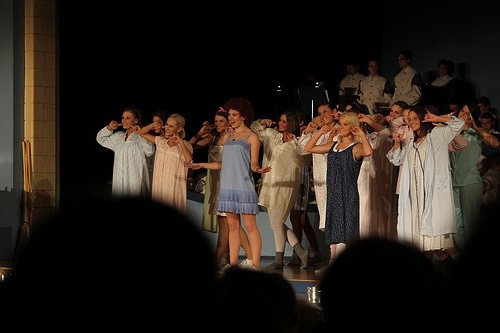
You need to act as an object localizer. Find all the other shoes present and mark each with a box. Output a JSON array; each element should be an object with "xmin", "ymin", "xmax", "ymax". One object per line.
[
  {"xmin": 238, "ymin": 259, "xmax": 245, "ymax": 266},
  {"xmin": 240, "ymin": 259, "xmax": 252, "ymax": 269},
  {"xmin": 217, "ymin": 264, "xmax": 231, "ymax": 275}
]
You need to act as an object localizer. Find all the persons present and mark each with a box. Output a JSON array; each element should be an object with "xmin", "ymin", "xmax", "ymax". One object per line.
[
  {"xmin": 449, "ymin": 103, "xmax": 500, "ymax": 252},
  {"xmin": 386, "ymin": 106, "xmax": 465, "ymax": 260},
  {"xmin": 189, "ymin": 107, "xmax": 231, "ymax": 267},
  {"xmin": 355, "ymin": 59, "xmax": 391, "ymax": 115},
  {"xmin": 427, "ymin": 59, "xmax": 470, "ymax": 114},
  {"xmin": 137, "ymin": 112, "xmax": 193, "ymax": 213},
  {"xmin": 250, "ymin": 111, "xmax": 311, "ymax": 270},
  {"xmin": 96, "ymin": 108, "xmax": 155, "ymax": 196},
  {"xmin": 317, "ymin": 201, "xmax": 500, "ymax": 333},
  {"xmin": 191, "ymin": 99, "xmax": 271, "ymax": 276},
  {"xmin": 344, "ymin": 101, "xmax": 414, "ymax": 240},
  {"xmin": 339, "ymin": 62, "xmax": 366, "ymax": 96},
  {"xmin": 212, "ymin": 268, "xmax": 295, "ymax": 333},
  {"xmin": 423, "ymin": 96, "xmax": 500, "ymax": 159},
  {"xmin": 305, "ymin": 112, "xmax": 373, "ymax": 256},
  {"xmin": 16, "ymin": 196, "xmax": 211, "ymax": 333},
  {"xmin": 390, "ymin": 51, "xmax": 422, "ymax": 108},
  {"xmin": 297, "ymin": 102, "xmax": 344, "ymax": 273}
]
